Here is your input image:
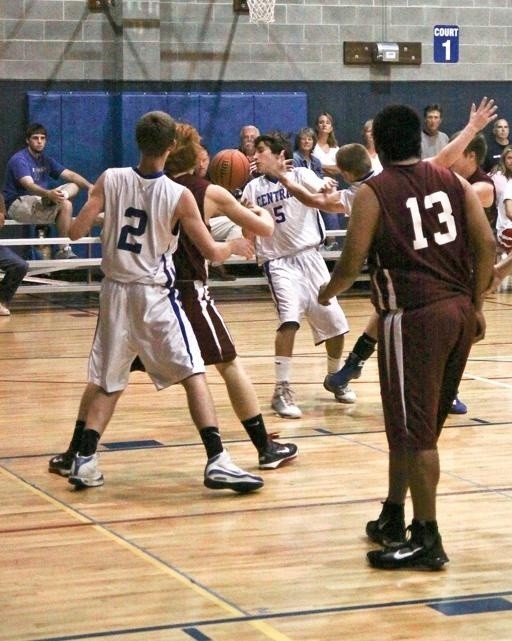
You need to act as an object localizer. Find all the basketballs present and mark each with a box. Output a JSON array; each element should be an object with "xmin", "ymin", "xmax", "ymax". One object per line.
[{"xmin": 209, "ymin": 149, "xmax": 251, "ymax": 192}]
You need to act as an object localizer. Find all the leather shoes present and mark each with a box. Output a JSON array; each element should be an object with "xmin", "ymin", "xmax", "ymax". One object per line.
[{"xmin": 210, "ymin": 264, "xmax": 236, "ymax": 280}]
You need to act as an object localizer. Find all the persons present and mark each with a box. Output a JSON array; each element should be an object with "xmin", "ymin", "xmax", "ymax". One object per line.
[
  {"xmin": 313, "ymin": 113, "xmax": 341, "ymax": 167},
  {"xmin": 491, "ymin": 147, "xmax": 512, "ymax": 201},
  {"xmin": 47, "ymin": 121, "xmax": 298, "ymax": 477},
  {"xmin": 363, "ymin": 118, "xmax": 383, "ymax": 176},
  {"xmin": 320, "ymin": 103, "xmax": 498, "ymax": 570},
  {"xmin": 271, "ymin": 96, "xmax": 499, "ymax": 413},
  {"xmin": 497, "ymin": 178, "xmax": 512, "ymax": 251},
  {"xmin": 240, "ymin": 135, "xmax": 357, "ymax": 419},
  {"xmin": 196, "ymin": 145, "xmax": 242, "ymax": 281},
  {"xmin": 239, "ymin": 125, "xmax": 261, "ymax": 156},
  {"xmin": 68, "ymin": 112, "xmax": 264, "ymax": 493},
  {"xmin": 5, "ymin": 124, "xmax": 94, "ymax": 259},
  {"xmin": 0, "ymin": 193, "xmax": 30, "ymax": 314},
  {"xmin": 324, "ymin": 128, "xmax": 497, "ymax": 386},
  {"xmin": 480, "ymin": 251, "xmax": 512, "ymax": 296},
  {"xmin": 484, "ymin": 119, "xmax": 510, "ymax": 169},
  {"xmin": 419, "ymin": 102, "xmax": 448, "ymax": 160},
  {"xmin": 293, "ymin": 127, "xmax": 339, "ymax": 249}
]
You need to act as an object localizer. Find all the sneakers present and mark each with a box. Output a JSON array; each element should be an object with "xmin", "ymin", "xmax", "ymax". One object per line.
[
  {"xmin": 272, "ymin": 386, "xmax": 302, "ymax": 418},
  {"xmin": 0, "ymin": 302, "xmax": 10, "ymax": 315},
  {"xmin": 58, "ymin": 245, "xmax": 77, "ymax": 258},
  {"xmin": 41, "ymin": 195, "xmax": 56, "ymax": 205},
  {"xmin": 367, "ymin": 535, "xmax": 449, "ymax": 571},
  {"xmin": 335, "ymin": 385, "xmax": 356, "ymax": 402},
  {"xmin": 49, "ymin": 455, "xmax": 72, "ymax": 475},
  {"xmin": 367, "ymin": 515, "xmax": 407, "ymax": 545},
  {"xmin": 323, "ymin": 359, "xmax": 362, "ymax": 390},
  {"xmin": 68, "ymin": 451, "xmax": 104, "ymax": 486},
  {"xmin": 449, "ymin": 397, "xmax": 468, "ymax": 413},
  {"xmin": 204, "ymin": 447, "xmax": 264, "ymax": 491},
  {"xmin": 259, "ymin": 443, "xmax": 298, "ymax": 467}
]
